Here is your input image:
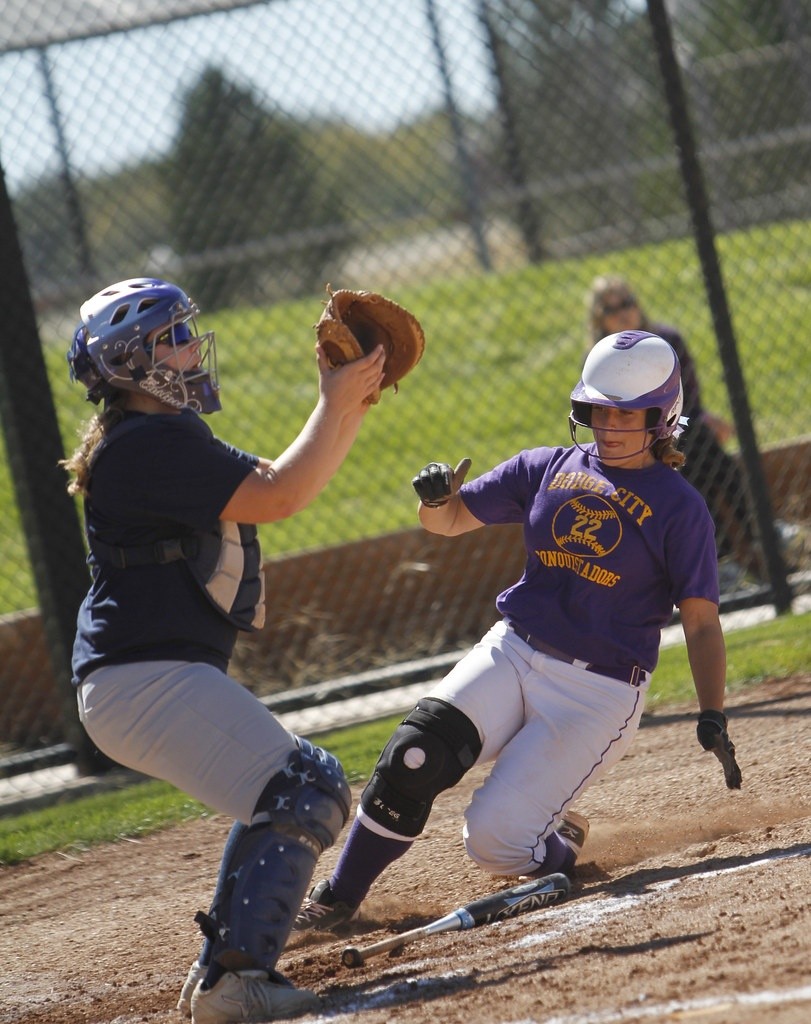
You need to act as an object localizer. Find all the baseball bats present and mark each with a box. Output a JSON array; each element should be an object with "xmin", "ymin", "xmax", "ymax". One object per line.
[{"xmin": 341, "ymin": 871, "xmax": 572, "ymax": 969}]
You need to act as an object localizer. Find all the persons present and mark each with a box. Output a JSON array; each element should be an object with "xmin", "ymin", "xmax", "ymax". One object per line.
[
  {"xmin": 283, "ymin": 329, "xmax": 744, "ymax": 952},
  {"xmin": 58, "ymin": 276, "xmax": 387, "ymax": 1023},
  {"xmin": 582, "ymin": 279, "xmax": 764, "ymax": 588}
]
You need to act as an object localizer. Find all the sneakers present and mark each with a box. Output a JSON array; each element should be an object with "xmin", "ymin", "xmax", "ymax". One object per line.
[
  {"xmin": 555, "ymin": 810, "xmax": 589, "ymax": 874},
  {"xmin": 191, "ymin": 963, "xmax": 321, "ymax": 1024},
  {"xmin": 176, "ymin": 958, "xmax": 208, "ymax": 1016},
  {"xmin": 278, "ymin": 878, "xmax": 361, "ymax": 948}
]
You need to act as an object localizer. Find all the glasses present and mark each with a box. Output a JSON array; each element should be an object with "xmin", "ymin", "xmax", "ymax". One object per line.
[
  {"xmin": 145, "ymin": 322, "xmax": 191, "ymax": 355},
  {"xmin": 597, "ymin": 295, "xmax": 636, "ymax": 321}
]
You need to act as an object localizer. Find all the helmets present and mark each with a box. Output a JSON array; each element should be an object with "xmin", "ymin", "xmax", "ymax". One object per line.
[
  {"xmin": 569, "ymin": 329, "xmax": 683, "ymax": 440},
  {"xmin": 67, "ymin": 277, "xmax": 222, "ymax": 414}
]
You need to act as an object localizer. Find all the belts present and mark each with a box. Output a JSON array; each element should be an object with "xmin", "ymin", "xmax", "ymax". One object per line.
[{"xmin": 510, "ymin": 624, "xmax": 647, "ymax": 689}]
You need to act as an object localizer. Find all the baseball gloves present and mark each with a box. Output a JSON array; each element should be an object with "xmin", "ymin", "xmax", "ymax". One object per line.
[{"xmin": 310, "ymin": 281, "xmax": 427, "ymax": 407}]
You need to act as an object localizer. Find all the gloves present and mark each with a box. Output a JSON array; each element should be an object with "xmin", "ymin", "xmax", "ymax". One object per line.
[
  {"xmin": 411, "ymin": 458, "xmax": 472, "ymax": 508},
  {"xmin": 697, "ymin": 709, "xmax": 743, "ymax": 790}
]
